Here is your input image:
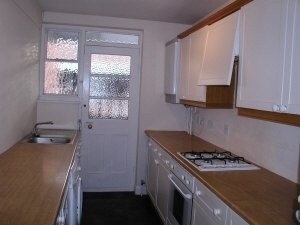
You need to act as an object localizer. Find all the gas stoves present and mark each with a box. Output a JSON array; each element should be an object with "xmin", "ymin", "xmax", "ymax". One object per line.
[{"xmin": 177, "ymin": 149, "xmax": 261, "ymax": 174}]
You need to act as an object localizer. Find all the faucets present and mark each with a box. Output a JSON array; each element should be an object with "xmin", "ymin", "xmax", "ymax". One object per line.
[{"xmin": 33, "ymin": 122, "xmax": 53, "ymax": 133}]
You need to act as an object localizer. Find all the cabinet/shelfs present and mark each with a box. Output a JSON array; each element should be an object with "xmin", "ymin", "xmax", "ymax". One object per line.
[
  {"xmin": 156, "ymin": 146, "xmax": 169, "ymax": 221},
  {"xmin": 178, "ymin": 16, "xmax": 237, "ymax": 109},
  {"xmin": 144, "ymin": 129, "xmax": 159, "ymax": 210},
  {"xmin": 191, "ymin": 177, "xmax": 226, "ymax": 225},
  {"xmin": 235, "ymin": 0, "xmax": 299, "ymax": 127},
  {"xmin": 164, "ymin": 38, "xmax": 179, "ymax": 104}
]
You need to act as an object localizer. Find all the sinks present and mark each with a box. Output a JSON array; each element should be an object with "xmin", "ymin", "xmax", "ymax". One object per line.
[{"xmin": 28, "ymin": 136, "xmax": 72, "ymax": 144}]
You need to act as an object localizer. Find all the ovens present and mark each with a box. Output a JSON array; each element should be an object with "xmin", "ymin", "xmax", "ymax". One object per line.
[{"xmin": 166, "ymin": 169, "xmax": 193, "ymax": 225}]
